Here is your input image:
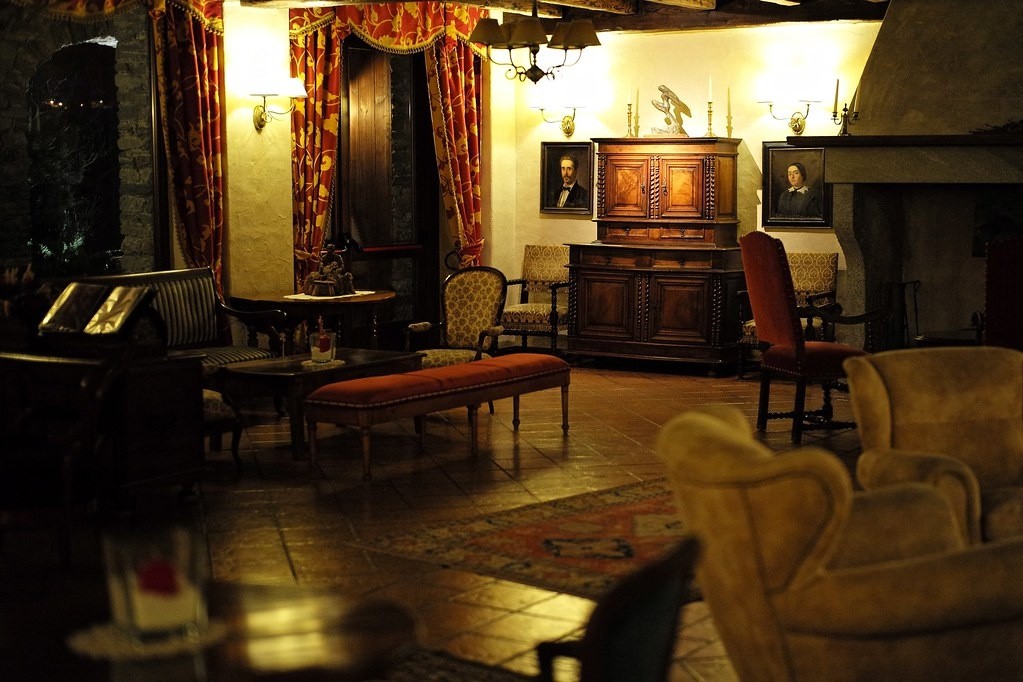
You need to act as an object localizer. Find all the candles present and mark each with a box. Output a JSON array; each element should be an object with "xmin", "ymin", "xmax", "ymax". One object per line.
[
  {"xmin": 853, "ymin": 75, "xmax": 862, "ymax": 112},
  {"xmin": 833, "ymin": 79, "xmax": 839, "ymax": 112},
  {"xmin": 627, "ymin": 85, "xmax": 631, "ymax": 104},
  {"xmin": 709, "ymin": 75, "xmax": 713, "ymax": 102}
]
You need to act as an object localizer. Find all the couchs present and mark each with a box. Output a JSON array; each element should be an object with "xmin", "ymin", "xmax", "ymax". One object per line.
[{"xmin": 653, "ymin": 343, "xmax": 1023, "ymax": 682}]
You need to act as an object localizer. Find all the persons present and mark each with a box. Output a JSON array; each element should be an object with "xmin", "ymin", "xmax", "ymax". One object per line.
[{"xmin": 320, "ymin": 243, "xmax": 344, "ymax": 270}]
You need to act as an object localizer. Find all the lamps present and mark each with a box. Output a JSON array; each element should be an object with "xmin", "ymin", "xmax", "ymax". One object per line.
[
  {"xmin": 532, "ymin": 104, "xmax": 586, "ymax": 137},
  {"xmin": 758, "ymin": 101, "xmax": 819, "ymax": 135},
  {"xmin": 469, "ymin": 1, "xmax": 601, "ymax": 82},
  {"xmin": 248, "ymin": 76, "xmax": 308, "ymax": 131}
]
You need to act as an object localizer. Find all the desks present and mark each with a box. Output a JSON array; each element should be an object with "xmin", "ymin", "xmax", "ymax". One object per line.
[
  {"xmin": 0, "ymin": 348, "xmax": 207, "ymax": 520},
  {"xmin": 0, "ymin": 565, "xmax": 427, "ymax": 682},
  {"xmin": 913, "ymin": 329, "xmax": 985, "ymax": 346},
  {"xmin": 229, "ymin": 286, "xmax": 398, "ymax": 354}
]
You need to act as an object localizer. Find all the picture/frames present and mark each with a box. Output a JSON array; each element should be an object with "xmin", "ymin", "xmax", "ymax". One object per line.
[
  {"xmin": 539, "ymin": 142, "xmax": 594, "ymax": 215},
  {"xmin": 762, "ymin": 141, "xmax": 833, "ymax": 229}
]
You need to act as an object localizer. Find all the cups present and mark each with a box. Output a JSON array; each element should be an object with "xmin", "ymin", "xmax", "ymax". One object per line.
[
  {"xmin": 309, "ymin": 331, "xmax": 336, "ymax": 362},
  {"xmin": 101, "ymin": 521, "xmax": 208, "ymax": 643}
]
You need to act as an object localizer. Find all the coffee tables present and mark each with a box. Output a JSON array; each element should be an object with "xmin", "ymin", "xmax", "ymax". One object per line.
[{"xmin": 210, "ymin": 348, "xmax": 427, "ymax": 462}]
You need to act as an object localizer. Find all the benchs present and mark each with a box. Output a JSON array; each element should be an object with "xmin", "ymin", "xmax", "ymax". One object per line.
[
  {"xmin": 304, "ymin": 352, "xmax": 570, "ymax": 482},
  {"xmin": 40, "ymin": 266, "xmax": 288, "ymax": 455}
]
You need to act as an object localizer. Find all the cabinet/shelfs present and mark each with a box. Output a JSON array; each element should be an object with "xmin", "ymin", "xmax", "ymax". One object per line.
[{"xmin": 562, "ymin": 138, "xmax": 753, "ymax": 377}]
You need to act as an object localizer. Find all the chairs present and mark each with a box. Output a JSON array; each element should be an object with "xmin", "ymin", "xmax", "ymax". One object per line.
[
  {"xmin": 736, "ymin": 251, "xmax": 885, "ymax": 445},
  {"xmin": 536, "ymin": 533, "xmax": 702, "ymax": 682},
  {"xmin": 403, "ymin": 244, "xmax": 570, "ymax": 419}
]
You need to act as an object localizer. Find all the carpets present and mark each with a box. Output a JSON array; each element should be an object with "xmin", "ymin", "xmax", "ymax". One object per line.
[
  {"xmin": 343, "ymin": 475, "xmax": 703, "ymax": 605},
  {"xmin": 388, "ymin": 644, "xmax": 533, "ymax": 682}
]
